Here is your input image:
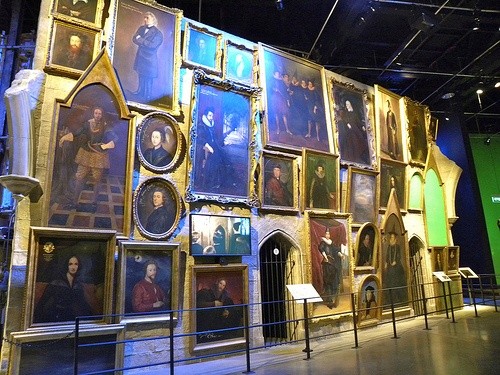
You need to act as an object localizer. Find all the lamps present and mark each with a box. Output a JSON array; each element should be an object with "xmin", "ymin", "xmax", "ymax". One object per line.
[
  {"xmin": 475, "ymin": 77, "xmax": 500, "ymax": 94},
  {"xmin": 471, "ymin": 7, "xmax": 481, "ymax": 30}
]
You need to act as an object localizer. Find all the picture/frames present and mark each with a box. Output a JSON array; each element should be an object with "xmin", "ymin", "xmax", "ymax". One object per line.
[{"xmin": 7, "ymin": 0, "xmax": 460, "ymax": 375}]
